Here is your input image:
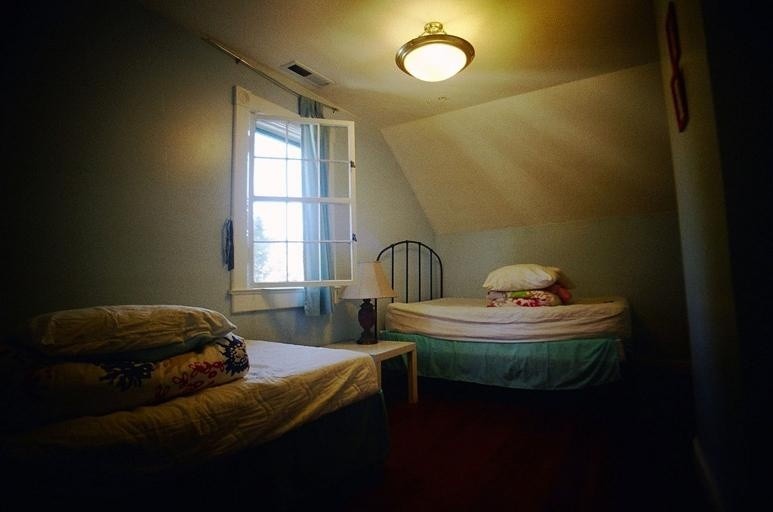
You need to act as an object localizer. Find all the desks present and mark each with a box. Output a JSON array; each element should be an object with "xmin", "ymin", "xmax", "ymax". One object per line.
[{"xmin": 319, "ymin": 338, "xmax": 419, "ymax": 407}]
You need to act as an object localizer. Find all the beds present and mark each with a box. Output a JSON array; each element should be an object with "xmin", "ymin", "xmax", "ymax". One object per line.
[
  {"xmin": 0, "ymin": 338, "xmax": 392, "ymax": 511},
  {"xmin": 371, "ymin": 240, "xmax": 635, "ymax": 396}
]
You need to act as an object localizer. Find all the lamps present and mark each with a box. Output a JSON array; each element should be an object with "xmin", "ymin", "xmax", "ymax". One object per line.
[
  {"xmin": 339, "ymin": 259, "xmax": 399, "ymax": 346},
  {"xmin": 395, "ymin": 21, "xmax": 475, "ymax": 84}
]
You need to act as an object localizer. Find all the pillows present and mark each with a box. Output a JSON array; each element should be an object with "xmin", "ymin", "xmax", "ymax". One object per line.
[
  {"xmin": 482, "ymin": 263, "xmax": 561, "ymax": 293},
  {"xmin": 24, "ymin": 301, "xmax": 238, "ymax": 362}
]
